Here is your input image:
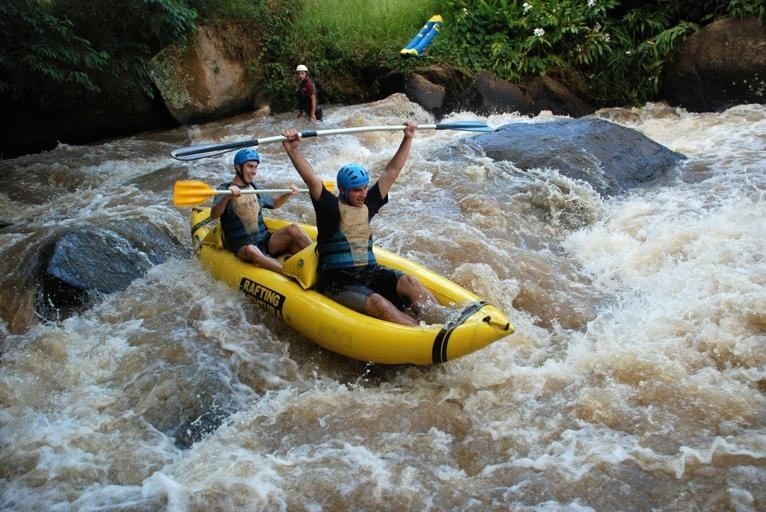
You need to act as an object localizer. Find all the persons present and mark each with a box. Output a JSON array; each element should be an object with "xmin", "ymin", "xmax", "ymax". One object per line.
[
  {"xmin": 280, "ymin": 122, "xmax": 449, "ymax": 328},
  {"xmin": 209, "ymin": 147, "xmax": 313, "ymax": 276},
  {"xmin": 294, "ymin": 61, "xmax": 324, "ymax": 123}
]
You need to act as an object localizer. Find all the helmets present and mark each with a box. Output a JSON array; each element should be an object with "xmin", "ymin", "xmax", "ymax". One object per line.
[
  {"xmin": 234, "ymin": 149, "xmax": 260, "ymax": 167},
  {"xmin": 296, "ymin": 65, "xmax": 308, "ymax": 72},
  {"xmin": 337, "ymin": 164, "xmax": 369, "ymax": 191}
]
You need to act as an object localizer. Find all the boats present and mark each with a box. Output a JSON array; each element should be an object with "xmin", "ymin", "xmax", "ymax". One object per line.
[
  {"xmin": 399, "ymin": 14, "xmax": 445, "ymax": 58},
  {"xmin": 191, "ymin": 204, "xmax": 516, "ymax": 366}
]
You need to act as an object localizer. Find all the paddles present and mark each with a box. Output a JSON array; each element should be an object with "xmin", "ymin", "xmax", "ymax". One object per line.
[
  {"xmin": 173, "ymin": 179, "xmax": 338, "ymax": 206},
  {"xmin": 170, "ymin": 121, "xmax": 493, "ymax": 162}
]
